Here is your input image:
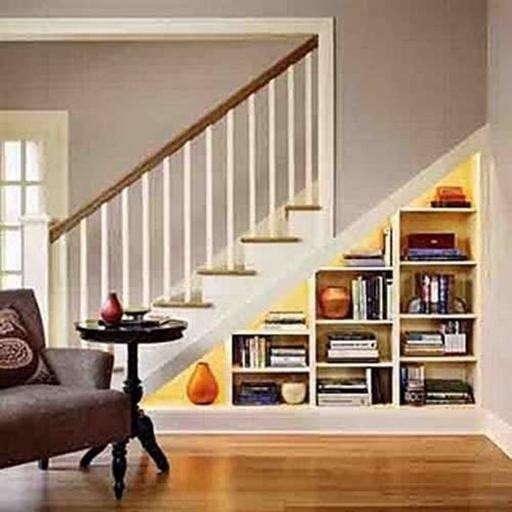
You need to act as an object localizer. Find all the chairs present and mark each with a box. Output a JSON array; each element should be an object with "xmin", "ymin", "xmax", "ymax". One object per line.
[{"xmin": 1, "ymin": 287, "xmax": 132, "ymax": 470}]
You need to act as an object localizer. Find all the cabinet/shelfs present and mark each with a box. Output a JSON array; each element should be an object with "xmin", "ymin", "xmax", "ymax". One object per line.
[{"xmin": 224, "ymin": 205, "xmax": 481, "ymax": 438}]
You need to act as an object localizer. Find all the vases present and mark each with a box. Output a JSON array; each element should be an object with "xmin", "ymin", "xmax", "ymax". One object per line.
[{"xmin": 187, "ymin": 362, "xmax": 218, "ymax": 405}]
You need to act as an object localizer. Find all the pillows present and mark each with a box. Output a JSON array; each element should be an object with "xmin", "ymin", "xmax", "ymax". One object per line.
[{"xmin": 0, "ymin": 304, "xmax": 62, "ymax": 388}]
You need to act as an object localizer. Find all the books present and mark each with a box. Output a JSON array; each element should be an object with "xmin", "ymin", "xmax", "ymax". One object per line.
[
  {"xmin": 400, "ymin": 183, "xmax": 480, "ymax": 407},
  {"xmin": 229, "ymin": 308, "xmax": 310, "ymax": 408},
  {"xmin": 315, "ymin": 225, "xmax": 395, "ymax": 407}
]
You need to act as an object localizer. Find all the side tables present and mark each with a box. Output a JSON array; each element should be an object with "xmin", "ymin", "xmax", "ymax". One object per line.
[{"xmin": 74, "ymin": 315, "xmax": 187, "ymax": 498}]
